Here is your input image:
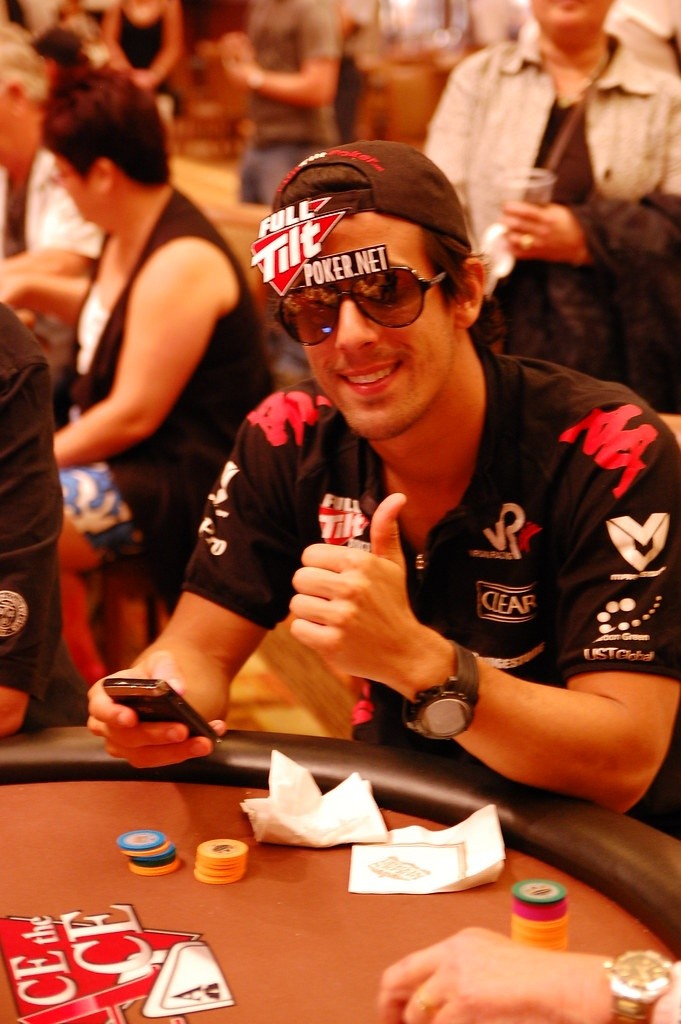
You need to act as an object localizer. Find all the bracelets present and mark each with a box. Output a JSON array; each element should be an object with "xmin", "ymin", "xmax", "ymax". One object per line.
[{"xmin": 247, "ymin": 70, "xmax": 264, "ymax": 91}]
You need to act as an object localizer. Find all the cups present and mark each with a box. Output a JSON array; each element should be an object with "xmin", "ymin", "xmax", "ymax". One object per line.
[{"xmin": 490, "ymin": 167, "xmax": 557, "ymax": 206}]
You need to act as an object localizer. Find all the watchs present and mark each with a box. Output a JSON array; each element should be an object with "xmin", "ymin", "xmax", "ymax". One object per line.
[
  {"xmin": 399, "ymin": 640, "xmax": 480, "ymax": 740},
  {"xmin": 599, "ymin": 951, "xmax": 673, "ymax": 1024}
]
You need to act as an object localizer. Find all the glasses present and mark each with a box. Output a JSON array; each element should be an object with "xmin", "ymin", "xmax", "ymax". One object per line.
[{"xmin": 274, "ymin": 262, "xmax": 449, "ymax": 346}]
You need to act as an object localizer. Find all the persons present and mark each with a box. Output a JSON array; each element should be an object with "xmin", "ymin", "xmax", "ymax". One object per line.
[
  {"xmin": 0, "ymin": 303, "xmax": 97, "ymax": 739},
  {"xmin": 3, "ymin": 44, "xmax": 103, "ymax": 261},
  {"xmin": 6, "ymin": 0, "xmax": 99, "ymax": 101},
  {"xmin": 419, "ymin": 0, "xmax": 680, "ymax": 419},
  {"xmin": 215, "ymin": 0, "xmax": 346, "ymax": 206},
  {"xmin": 87, "ymin": 139, "xmax": 680, "ymax": 842},
  {"xmin": 324, "ymin": 0, "xmax": 531, "ymax": 141},
  {"xmin": 1, "ymin": 68, "xmax": 279, "ymax": 695},
  {"xmin": 370, "ymin": 929, "xmax": 679, "ymax": 1024},
  {"xmin": 98, "ymin": 1, "xmax": 185, "ymax": 126}
]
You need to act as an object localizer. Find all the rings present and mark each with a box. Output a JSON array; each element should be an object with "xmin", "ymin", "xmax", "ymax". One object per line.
[{"xmin": 520, "ymin": 233, "xmax": 533, "ymax": 251}]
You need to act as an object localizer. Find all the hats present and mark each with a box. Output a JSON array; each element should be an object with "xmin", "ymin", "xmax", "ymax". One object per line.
[{"xmin": 269, "ymin": 140, "xmax": 472, "ymax": 252}]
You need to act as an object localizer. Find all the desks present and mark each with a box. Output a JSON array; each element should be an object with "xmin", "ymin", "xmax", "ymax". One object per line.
[{"xmin": 1, "ymin": 728, "xmax": 681, "ymax": 1023}]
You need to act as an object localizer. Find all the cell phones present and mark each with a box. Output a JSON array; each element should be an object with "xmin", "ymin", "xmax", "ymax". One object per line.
[{"xmin": 101, "ymin": 676, "xmax": 218, "ymax": 751}]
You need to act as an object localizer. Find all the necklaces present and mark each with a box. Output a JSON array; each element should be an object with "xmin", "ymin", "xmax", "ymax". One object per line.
[{"xmin": 547, "ymin": 43, "xmax": 610, "ymax": 108}]
